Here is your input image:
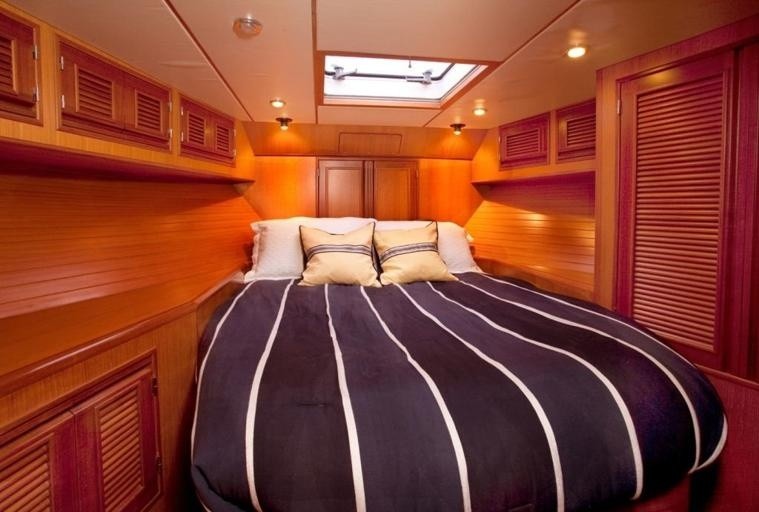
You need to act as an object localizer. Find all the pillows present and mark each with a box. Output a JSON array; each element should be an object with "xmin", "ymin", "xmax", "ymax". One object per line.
[
  {"xmin": 375, "ymin": 220, "xmax": 487, "ymax": 276},
  {"xmin": 373, "ymin": 220, "xmax": 461, "ymax": 286},
  {"xmin": 300, "ymin": 222, "xmax": 379, "ymax": 288},
  {"xmin": 245, "ymin": 218, "xmax": 379, "ymax": 288}
]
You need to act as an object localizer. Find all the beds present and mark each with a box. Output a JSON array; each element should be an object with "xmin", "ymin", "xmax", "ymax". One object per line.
[{"xmin": 185, "ymin": 221, "xmax": 728, "ymax": 509}]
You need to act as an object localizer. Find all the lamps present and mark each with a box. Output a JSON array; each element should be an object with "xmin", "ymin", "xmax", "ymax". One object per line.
[
  {"xmin": 450, "ymin": 122, "xmax": 466, "ymax": 137},
  {"xmin": 276, "ymin": 117, "xmax": 292, "ymax": 130}
]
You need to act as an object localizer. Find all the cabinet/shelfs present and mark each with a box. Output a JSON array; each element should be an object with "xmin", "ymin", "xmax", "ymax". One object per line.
[
  {"xmin": 615, "ymin": 40, "xmax": 758, "ymax": 384},
  {"xmin": 178, "ymin": 94, "xmax": 239, "ymax": 168},
  {"xmin": 555, "ymin": 99, "xmax": 596, "ymax": 165},
  {"xmin": 59, "ymin": 39, "xmax": 172, "ymax": 149},
  {"xmin": 317, "ymin": 157, "xmax": 419, "ymax": 220},
  {"xmin": 0, "ymin": 12, "xmax": 42, "ymax": 127},
  {"xmin": 498, "ymin": 110, "xmax": 550, "ymax": 171},
  {"xmin": 1, "ymin": 350, "xmax": 164, "ymax": 511}
]
